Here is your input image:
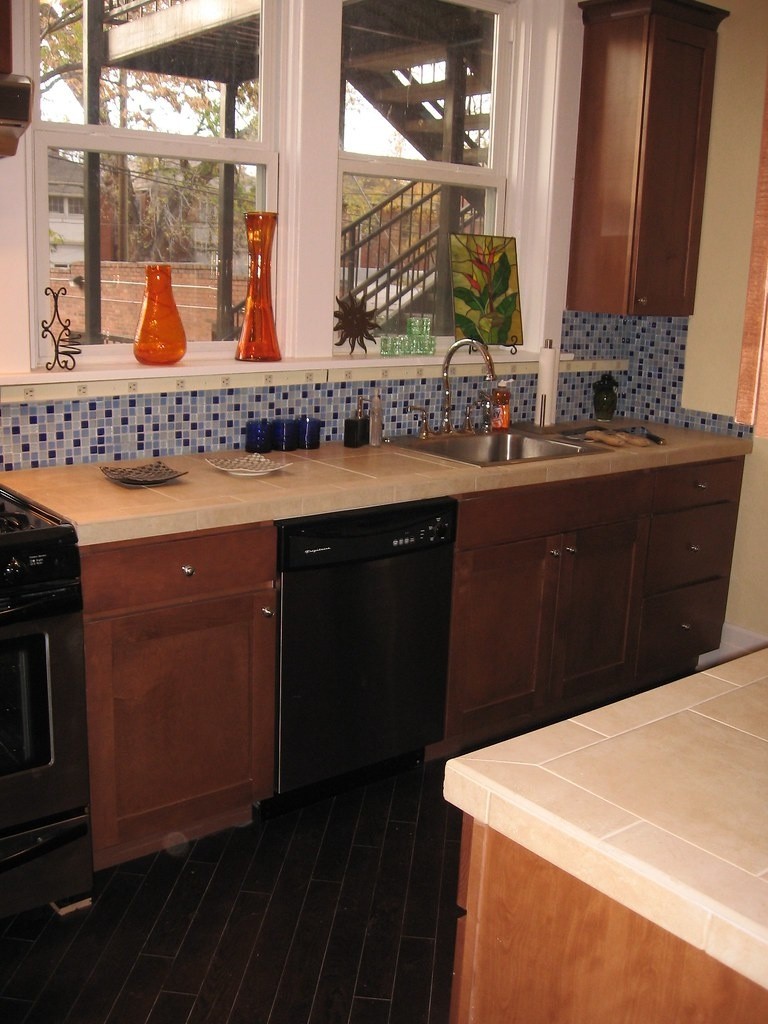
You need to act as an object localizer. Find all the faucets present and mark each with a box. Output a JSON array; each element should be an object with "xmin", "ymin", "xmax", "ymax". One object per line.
[{"xmin": 437, "ymin": 339, "xmax": 498, "ymax": 439}]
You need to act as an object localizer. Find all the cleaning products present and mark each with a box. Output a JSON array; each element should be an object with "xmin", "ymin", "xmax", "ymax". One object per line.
[{"xmin": 492, "ymin": 379, "xmax": 515, "ymax": 432}]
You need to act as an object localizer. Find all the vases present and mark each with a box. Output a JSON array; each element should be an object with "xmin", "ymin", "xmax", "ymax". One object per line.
[
  {"xmin": 133, "ymin": 265, "xmax": 186, "ymax": 366},
  {"xmin": 236, "ymin": 211, "xmax": 281, "ymax": 363}
]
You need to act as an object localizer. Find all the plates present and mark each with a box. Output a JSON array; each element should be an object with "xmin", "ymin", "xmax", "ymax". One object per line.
[
  {"xmin": 205, "ymin": 452, "xmax": 293, "ymax": 476},
  {"xmin": 99, "ymin": 460, "xmax": 189, "ymax": 487}
]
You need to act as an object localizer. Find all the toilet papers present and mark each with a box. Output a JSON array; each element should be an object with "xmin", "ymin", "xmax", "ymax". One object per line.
[{"xmin": 532, "ymin": 345, "xmax": 561, "ymax": 426}]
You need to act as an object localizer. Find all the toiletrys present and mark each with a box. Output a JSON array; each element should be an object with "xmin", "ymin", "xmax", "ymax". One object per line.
[
  {"xmin": 343, "ymin": 396, "xmax": 373, "ymax": 446},
  {"xmin": 368, "ymin": 388, "xmax": 383, "ymax": 447}
]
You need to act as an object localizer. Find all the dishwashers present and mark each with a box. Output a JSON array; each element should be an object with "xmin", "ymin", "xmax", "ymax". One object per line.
[{"xmin": 269, "ymin": 494, "xmax": 458, "ymax": 799}]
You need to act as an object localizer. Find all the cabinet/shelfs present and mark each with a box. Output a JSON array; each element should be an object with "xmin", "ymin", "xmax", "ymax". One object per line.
[
  {"xmin": 79, "ymin": 520, "xmax": 275, "ymax": 850},
  {"xmin": 635, "ymin": 455, "xmax": 746, "ymax": 680},
  {"xmin": 566, "ymin": 15, "xmax": 718, "ymax": 314},
  {"xmin": 444, "ymin": 469, "xmax": 654, "ymax": 739}
]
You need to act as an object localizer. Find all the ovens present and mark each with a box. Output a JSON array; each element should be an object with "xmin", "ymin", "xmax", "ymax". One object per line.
[{"xmin": 0, "ymin": 586, "xmax": 94, "ymax": 914}]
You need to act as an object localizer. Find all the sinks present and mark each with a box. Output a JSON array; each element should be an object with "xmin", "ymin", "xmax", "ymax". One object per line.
[{"xmin": 377, "ymin": 428, "xmax": 615, "ymax": 469}]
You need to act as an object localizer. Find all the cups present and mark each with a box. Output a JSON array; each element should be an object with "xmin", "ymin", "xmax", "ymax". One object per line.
[
  {"xmin": 245, "ymin": 421, "xmax": 273, "ymax": 452},
  {"xmin": 272, "ymin": 419, "xmax": 300, "ymax": 451},
  {"xmin": 380, "ymin": 317, "xmax": 436, "ymax": 357},
  {"xmin": 297, "ymin": 417, "xmax": 321, "ymax": 450}
]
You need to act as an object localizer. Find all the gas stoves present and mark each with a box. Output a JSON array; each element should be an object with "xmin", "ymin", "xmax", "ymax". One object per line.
[{"xmin": 0, "ymin": 482, "xmax": 81, "ymax": 588}]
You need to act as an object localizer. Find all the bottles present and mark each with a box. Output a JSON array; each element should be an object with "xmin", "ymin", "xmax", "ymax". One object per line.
[
  {"xmin": 534, "ymin": 339, "xmax": 556, "ymax": 427},
  {"xmin": 594, "ymin": 374, "xmax": 618, "ymax": 422},
  {"xmin": 235, "ymin": 212, "xmax": 282, "ymax": 362},
  {"xmin": 133, "ymin": 264, "xmax": 187, "ymax": 366}
]
